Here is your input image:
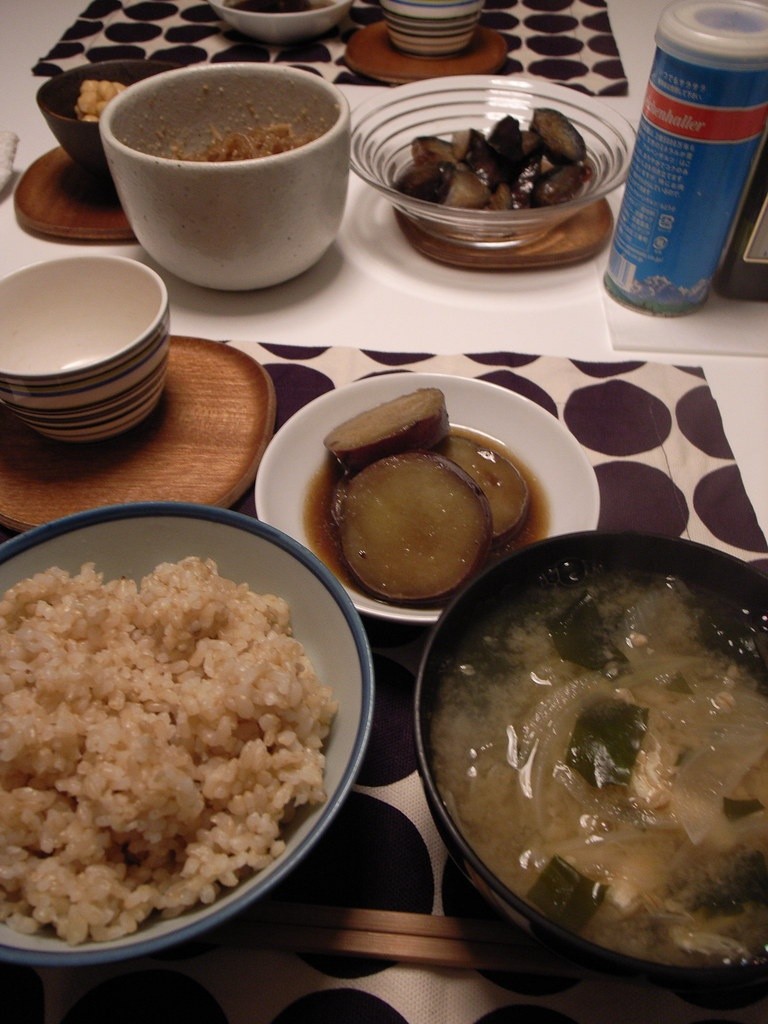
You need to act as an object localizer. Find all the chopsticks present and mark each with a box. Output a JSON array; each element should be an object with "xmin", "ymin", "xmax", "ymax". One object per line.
[{"xmin": 209, "ymin": 902, "xmax": 584, "ymax": 977}]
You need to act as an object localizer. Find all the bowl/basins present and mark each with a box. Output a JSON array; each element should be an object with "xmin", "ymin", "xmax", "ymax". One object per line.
[
  {"xmin": 254, "ymin": 372, "xmax": 601, "ymax": 624},
  {"xmin": 351, "ymin": 74, "xmax": 638, "ymax": 249},
  {"xmin": 412, "ymin": 529, "xmax": 768, "ymax": 995},
  {"xmin": 0, "ymin": 501, "xmax": 374, "ymax": 967},
  {"xmin": 37, "ymin": 59, "xmax": 190, "ymax": 191},
  {"xmin": 101, "ymin": 62, "xmax": 351, "ymax": 291},
  {"xmin": 207, "ymin": 0, "xmax": 355, "ymax": 43},
  {"xmin": 0, "ymin": 253, "xmax": 171, "ymax": 445}
]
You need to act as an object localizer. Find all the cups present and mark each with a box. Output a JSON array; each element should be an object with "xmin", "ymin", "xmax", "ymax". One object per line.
[{"xmin": 379, "ymin": 0, "xmax": 485, "ymax": 58}]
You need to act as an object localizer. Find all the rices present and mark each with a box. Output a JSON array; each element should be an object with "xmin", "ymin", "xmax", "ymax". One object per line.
[{"xmin": 0, "ymin": 554, "xmax": 340, "ymax": 944}]
